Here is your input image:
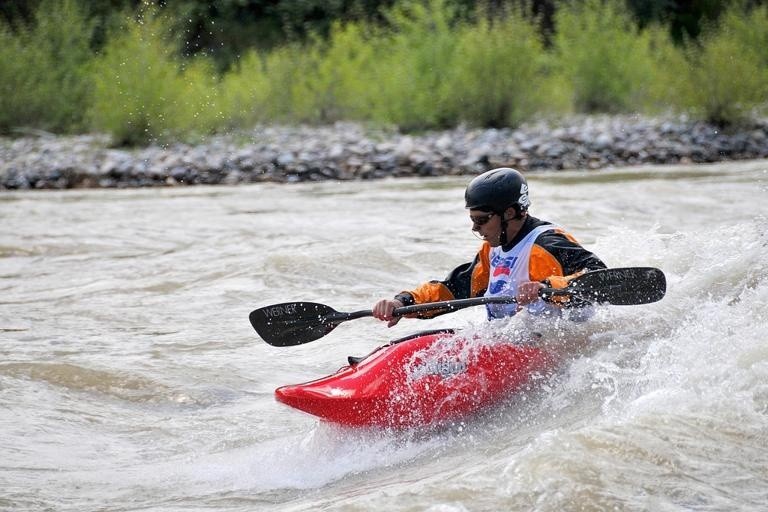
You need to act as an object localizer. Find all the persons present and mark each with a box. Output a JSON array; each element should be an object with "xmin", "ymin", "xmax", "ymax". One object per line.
[{"xmin": 347, "ymin": 168, "xmax": 606, "ymax": 370}]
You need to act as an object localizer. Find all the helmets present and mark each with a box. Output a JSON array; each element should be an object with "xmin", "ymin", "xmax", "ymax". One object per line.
[{"xmin": 464, "ymin": 167, "xmax": 531, "ymax": 213}]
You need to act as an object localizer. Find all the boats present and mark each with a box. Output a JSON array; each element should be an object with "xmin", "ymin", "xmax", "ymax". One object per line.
[{"xmin": 276, "ymin": 329, "xmax": 556, "ymax": 426}]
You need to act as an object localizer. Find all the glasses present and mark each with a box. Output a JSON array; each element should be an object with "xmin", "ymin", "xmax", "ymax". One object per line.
[{"xmin": 470, "ymin": 213, "xmax": 496, "ymax": 225}]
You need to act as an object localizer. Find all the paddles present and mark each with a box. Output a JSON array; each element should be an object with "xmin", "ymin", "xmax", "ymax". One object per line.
[{"xmin": 249, "ymin": 266, "xmax": 666, "ymax": 347}]
